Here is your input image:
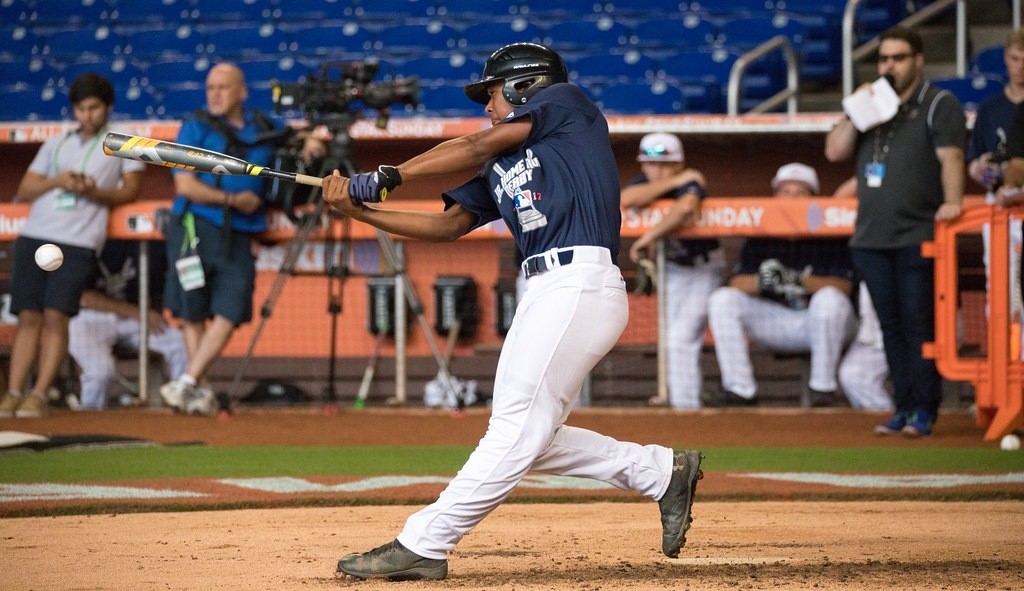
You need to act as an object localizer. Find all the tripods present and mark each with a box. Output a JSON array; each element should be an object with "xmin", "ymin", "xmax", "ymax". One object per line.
[{"xmin": 223, "ymin": 114, "xmax": 469, "ymax": 411}]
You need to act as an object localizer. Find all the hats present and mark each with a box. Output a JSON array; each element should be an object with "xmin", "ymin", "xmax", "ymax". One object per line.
[
  {"xmin": 636, "ymin": 132, "xmax": 685, "ymax": 165},
  {"xmin": 774, "ymin": 162, "xmax": 821, "ymax": 196}
]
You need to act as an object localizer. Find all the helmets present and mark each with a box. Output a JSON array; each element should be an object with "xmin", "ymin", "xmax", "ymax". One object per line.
[{"xmin": 464, "ymin": 42, "xmax": 567, "ymax": 107}]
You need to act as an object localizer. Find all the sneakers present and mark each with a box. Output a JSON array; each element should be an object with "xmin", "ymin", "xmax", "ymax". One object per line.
[
  {"xmin": 658, "ymin": 448, "xmax": 706, "ymax": 558},
  {"xmin": 337, "ymin": 539, "xmax": 448, "ymax": 581},
  {"xmin": 901, "ymin": 409, "xmax": 934, "ymax": 439},
  {"xmin": 872, "ymin": 410, "xmax": 911, "ymax": 436}
]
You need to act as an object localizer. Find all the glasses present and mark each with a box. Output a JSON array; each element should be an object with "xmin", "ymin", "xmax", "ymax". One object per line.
[
  {"xmin": 875, "ymin": 51, "xmax": 915, "ymax": 63},
  {"xmin": 639, "ymin": 147, "xmax": 675, "ymax": 156}
]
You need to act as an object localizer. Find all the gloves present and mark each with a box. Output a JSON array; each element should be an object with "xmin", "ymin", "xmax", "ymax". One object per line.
[
  {"xmin": 759, "ymin": 259, "xmax": 784, "ymax": 291},
  {"xmin": 776, "ymin": 269, "xmax": 804, "ymax": 295},
  {"xmin": 968, "ymin": 153, "xmax": 1000, "ymax": 191},
  {"xmin": 349, "ymin": 164, "xmax": 402, "ymax": 204}
]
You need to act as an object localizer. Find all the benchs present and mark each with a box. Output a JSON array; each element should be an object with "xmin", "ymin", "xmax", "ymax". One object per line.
[{"xmin": 575, "ymin": 295, "xmax": 814, "ymax": 405}]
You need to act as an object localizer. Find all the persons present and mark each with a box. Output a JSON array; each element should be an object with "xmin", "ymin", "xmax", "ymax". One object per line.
[
  {"xmin": 708, "ymin": 162, "xmax": 858, "ymax": 410},
  {"xmin": 65, "ymin": 238, "xmax": 189, "ymax": 411},
  {"xmin": 618, "ymin": 129, "xmax": 724, "ymax": 411},
  {"xmin": 824, "ymin": 26, "xmax": 967, "ymax": 437},
  {"xmin": 967, "ymin": 34, "xmax": 1023, "ymax": 450},
  {"xmin": 0, "ymin": 72, "xmax": 146, "ymax": 419},
  {"xmin": 834, "ymin": 173, "xmax": 897, "ymax": 412},
  {"xmin": 156, "ymin": 62, "xmax": 282, "ymax": 417},
  {"xmin": 321, "ymin": 42, "xmax": 705, "ymax": 579}
]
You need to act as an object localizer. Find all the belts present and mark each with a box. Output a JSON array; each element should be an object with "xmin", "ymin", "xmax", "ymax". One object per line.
[{"xmin": 522, "ymin": 248, "xmax": 621, "ymax": 279}]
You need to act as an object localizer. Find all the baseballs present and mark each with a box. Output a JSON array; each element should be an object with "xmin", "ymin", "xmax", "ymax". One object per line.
[
  {"xmin": 34, "ymin": 243, "xmax": 65, "ymax": 272},
  {"xmin": 1000, "ymin": 434, "xmax": 1021, "ymax": 450}
]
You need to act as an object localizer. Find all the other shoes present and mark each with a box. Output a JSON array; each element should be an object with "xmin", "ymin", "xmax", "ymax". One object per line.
[
  {"xmin": 15, "ymin": 391, "xmax": 48, "ymax": 418},
  {"xmin": 698, "ymin": 388, "xmax": 761, "ymax": 408},
  {"xmin": 186, "ymin": 387, "xmax": 220, "ymax": 417},
  {"xmin": 806, "ymin": 386, "xmax": 836, "ymax": 407},
  {"xmin": 0, "ymin": 394, "xmax": 22, "ymax": 418},
  {"xmin": 159, "ymin": 380, "xmax": 192, "ymax": 413}
]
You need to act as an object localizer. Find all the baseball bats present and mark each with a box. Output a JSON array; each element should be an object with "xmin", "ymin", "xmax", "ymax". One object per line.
[
  {"xmin": 102, "ymin": 130, "xmax": 389, "ymax": 203},
  {"xmin": 354, "ymin": 323, "xmax": 390, "ymax": 409}
]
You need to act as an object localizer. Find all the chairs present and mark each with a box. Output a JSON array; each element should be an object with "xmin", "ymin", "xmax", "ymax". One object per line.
[{"xmin": 0, "ymin": 0, "xmax": 1013, "ymax": 124}]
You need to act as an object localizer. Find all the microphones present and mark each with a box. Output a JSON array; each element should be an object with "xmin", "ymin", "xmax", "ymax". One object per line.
[{"xmin": 847, "ymin": 74, "xmax": 896, "ymax": 119}]
